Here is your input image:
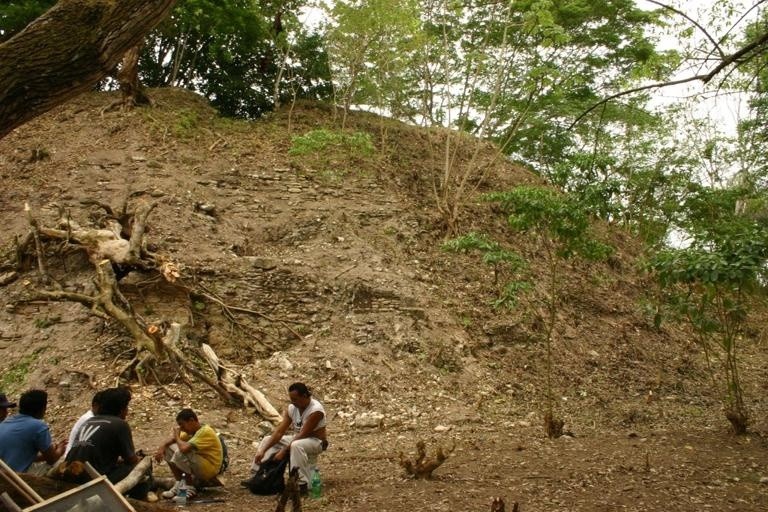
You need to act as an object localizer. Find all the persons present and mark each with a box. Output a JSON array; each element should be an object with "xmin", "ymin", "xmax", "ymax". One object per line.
[
  {"xmin": 153, "ymin": 408, "xmax": 224, "ymax": 503},
  {"xmin": 0, "ymin": 389, "xmax": 69, "ymax": 474},
  {"xmin": 62, "ymin": 390, "xmax": 102, "ymax": 459},
  {"xmin": 0, "ymin": 393, "xmax": 16, "ymax": 423},
  {"xmin": 238, "ymin": 382, "xmax": 329, "ymax": 497},
  {"xmin": 70, "ymin": 385, "xmax": 159, "ymax": 503}
]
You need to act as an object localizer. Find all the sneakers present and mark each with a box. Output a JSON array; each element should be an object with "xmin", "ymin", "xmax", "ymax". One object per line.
[
  {"xmin": 162, "ymin": 481, "xmax": 181, "ymax": 498},
  {"xmin": 240, "ymin": 475, "xmax": 254, "ymax": 487},
  {"xmin": 299, "ymin": 482, "xmax": 310, "ymax": 497},
  {"xmin": 172, "ymin": 486, "xmax": 199, "ymax": 503}
]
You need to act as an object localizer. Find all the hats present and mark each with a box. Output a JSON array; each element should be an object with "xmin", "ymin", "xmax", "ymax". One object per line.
[{"xmin": 1, "ymin": 393, "xmax": 18, "ymax": 409}]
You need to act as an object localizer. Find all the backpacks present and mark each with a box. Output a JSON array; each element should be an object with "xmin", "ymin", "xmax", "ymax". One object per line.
[
  {"xmin": 216, "ymin": 432, "xmax": 230, "ymax": 475},
  {"xmin": 127, "ymin": 448, "xmax": 155, "ymax": 499},
  {"xmin": 251, "ymin": 450, "xmax": 289, "ymax": 496}
]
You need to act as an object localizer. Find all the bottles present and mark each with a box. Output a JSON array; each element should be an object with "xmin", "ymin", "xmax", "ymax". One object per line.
[
  {"xmin": 311, "ymin": 469, "xmax": 322, "ymax": 499},
  {"xmin": 176, "ymin": 473, "xmax": 187, "ymax": 507}
]
